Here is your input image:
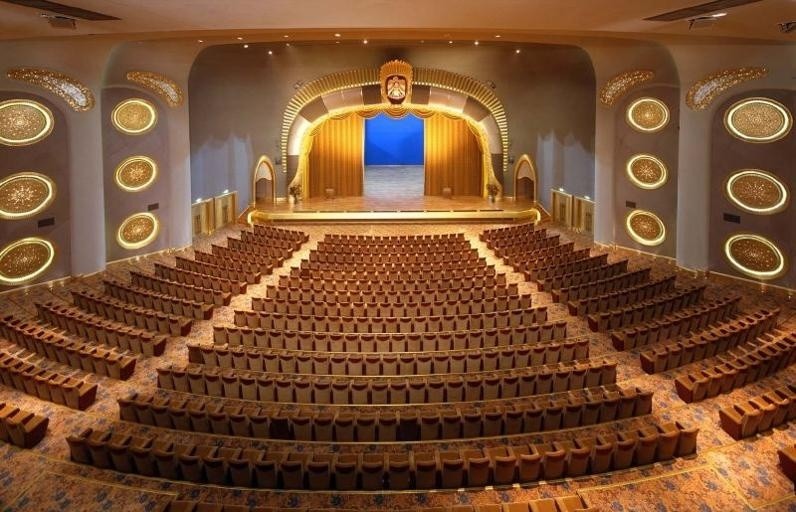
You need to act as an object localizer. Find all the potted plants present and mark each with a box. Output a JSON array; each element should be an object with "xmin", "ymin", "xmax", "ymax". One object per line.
[
  {"xmin": 487, "ymin": 183, "xmax": 499, "ymax": 204},
  {"xmin": 290, "ymin": 184, "xmax": 302, "ymax": 204}
]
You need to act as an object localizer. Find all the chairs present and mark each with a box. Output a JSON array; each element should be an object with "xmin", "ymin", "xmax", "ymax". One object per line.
[
  {"xmin": 169, "ymin": 498, "xmax": 280, "ymax": 511},
  {"xmin": 431, "ymin": 493, "xmax": 601, "ymax": 512},
  {"xmin": 2, "ymin": 223, "xmax": 796, "ymax": 490}
]
